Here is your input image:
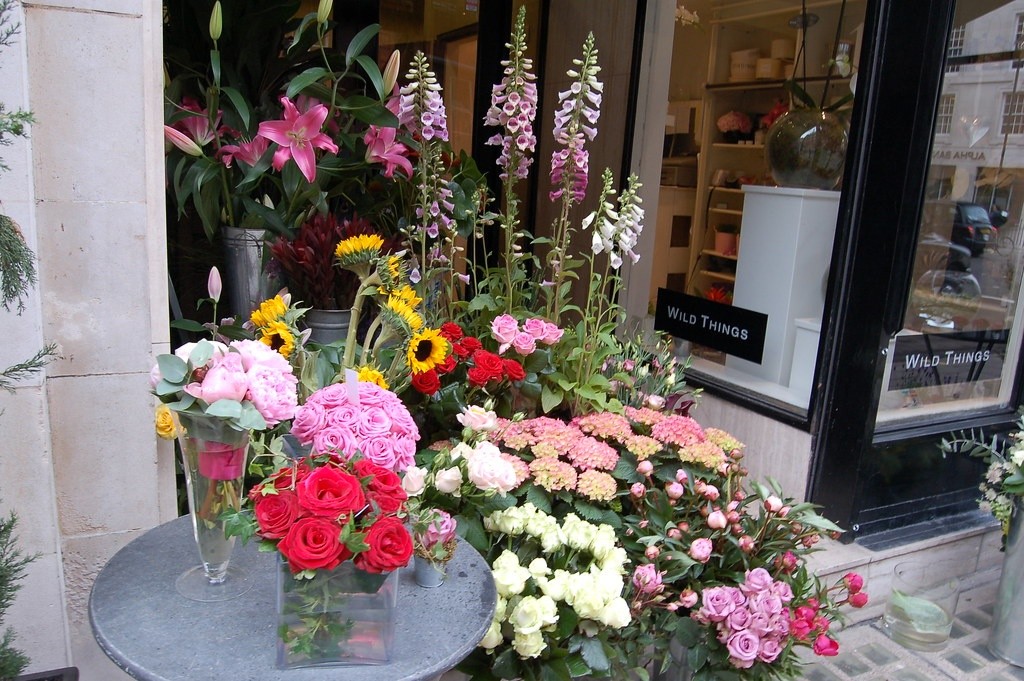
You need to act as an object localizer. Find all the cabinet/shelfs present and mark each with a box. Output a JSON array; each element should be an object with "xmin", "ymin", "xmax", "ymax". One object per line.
[{"xmin": 685, "ymin": 0, "xmax": 867, "ymax": 296}]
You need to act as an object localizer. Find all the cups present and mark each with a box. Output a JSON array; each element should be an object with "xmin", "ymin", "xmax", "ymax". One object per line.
[{"xmin": 881, "ymin": 560, "xmax": 962, "ymax": 652}]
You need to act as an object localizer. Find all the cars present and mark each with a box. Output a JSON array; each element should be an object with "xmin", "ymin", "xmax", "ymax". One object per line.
[{"xmin": 951, "ymin": 201, "xmax": 997, "ymax": 258}]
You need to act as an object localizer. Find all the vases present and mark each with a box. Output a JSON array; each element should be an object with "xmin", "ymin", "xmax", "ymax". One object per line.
[
  {"xmin": 985, "ymin": 506, "xmax": 1024, "ymax": 669},
  {"xmin": 275, "ymin": 548, "xmax": 399, "ymax": 669},
  {"xmin": 414, "ymin": 553, "xmax": 445, "ymax": 588},
  {"xmin": 221, "ymin": 225, "xmax": 300, "ymax": 323},
  {"xmin": 168, "ymin": 406, "xmax": 255, "ymax": 602},
  {"xmin": 298, "ymin": 309, "xmax": 371, "ymax": 344},
  {"xmin": 883, "ymin": 561, "xmax": 959, "ymax": 652}
]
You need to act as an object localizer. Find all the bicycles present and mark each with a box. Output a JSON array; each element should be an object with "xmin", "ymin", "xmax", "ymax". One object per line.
[{"xmin": 984, "ymin": 226, "xmax": 1015, "ymax": 257}]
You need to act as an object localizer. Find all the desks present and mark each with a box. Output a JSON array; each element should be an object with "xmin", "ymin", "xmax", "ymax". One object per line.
[{"xmin": 88, "ymin": 508, "xmax": 497, "ymax": 680}]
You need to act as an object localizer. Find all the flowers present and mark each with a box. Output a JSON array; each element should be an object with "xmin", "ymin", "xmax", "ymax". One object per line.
[
  {"xmin": 163, "ymin": 1, "xmax": 412, "ymax": 245},
  {"xmin": 935, "ymin": 405, "xmax": 1024, "ymax": 552},
  {"xmin": 147, "ymin": 339, "xmax": 297, "ymax": 439},
  {"xmin": 264, "ymin": 208, "xmax": 399, "ymax": 310},
  {"xmin": 717, "ymin": 110, "xmax": 753, "ymax": 134},
  {"xmin": 215, "ymin": 380, "xmax": 461, "ymax": 658}
]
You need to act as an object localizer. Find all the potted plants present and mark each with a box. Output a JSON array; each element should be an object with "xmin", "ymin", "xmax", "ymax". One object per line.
[
  {"xmin": 0, "ymin": 0, "xmax": 80, "ymax": 681},
  {"xmin": 764, "ymin": 80, "xmax": 855, "ymax": 189},
  {"xmin": 711, "ymin": 223, "xmax": 741, "ymax": 255}
]
